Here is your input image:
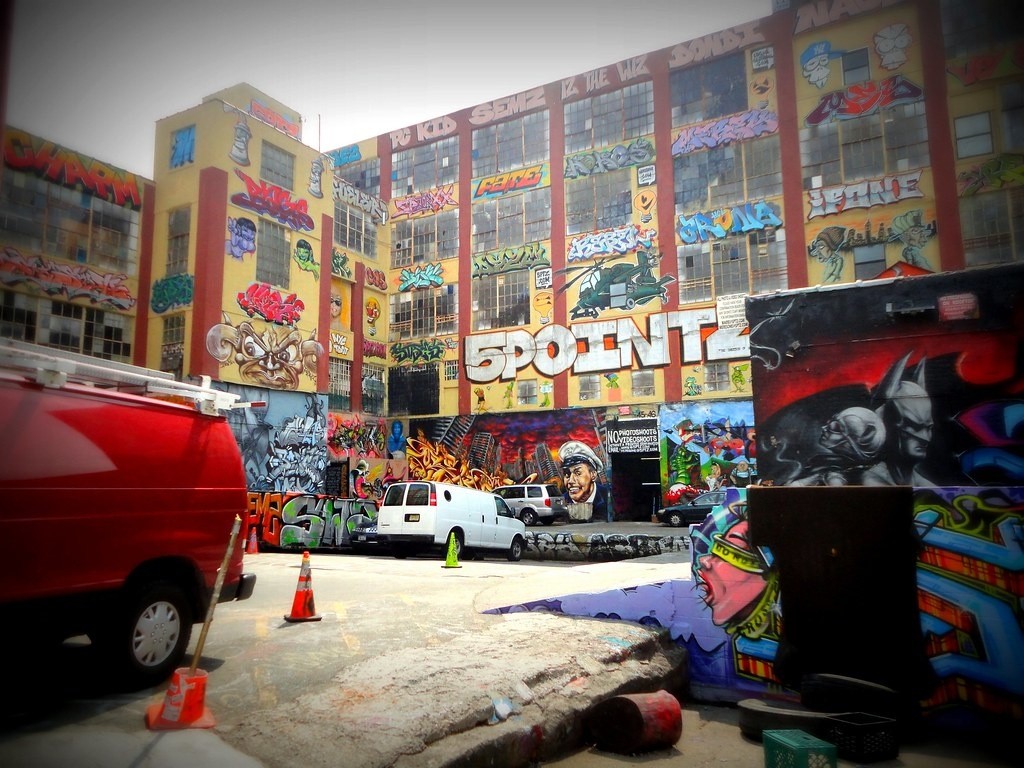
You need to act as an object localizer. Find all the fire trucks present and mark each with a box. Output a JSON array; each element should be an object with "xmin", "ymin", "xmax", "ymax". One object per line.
[{"xmin": 0, "ymin": 333, "xmax": 260, "ymax": 688}]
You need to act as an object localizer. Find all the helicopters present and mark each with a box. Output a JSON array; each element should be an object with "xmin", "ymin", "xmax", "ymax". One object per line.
[{"xmin": 550, "ymin": 248, "xmax": 678, "ymax": 321}]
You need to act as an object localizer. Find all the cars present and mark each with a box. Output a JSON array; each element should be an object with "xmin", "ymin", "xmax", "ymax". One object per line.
[
  {"xmin": 656, "ymin": 490, "xmax": 732, "ymax": 527},
  {"xmin": 348, "ymin": 520, "xmax": 417, "ymax": 558}
]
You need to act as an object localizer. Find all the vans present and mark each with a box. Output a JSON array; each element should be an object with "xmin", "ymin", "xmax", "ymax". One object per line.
[
  {"xmin": 483, "ymin": 481, "xmax": 568, "ymax": 526},
  {"xmin": 375, "ymin": 477, "xmax": 527, "ymax": 562}
]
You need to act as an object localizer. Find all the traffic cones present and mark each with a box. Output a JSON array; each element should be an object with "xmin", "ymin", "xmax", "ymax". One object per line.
[
  {"xmin": 441, "ymin": 531, "xmax": 464, "ymax": 568},
  {"xmin": 283, "ymin": 550, "xmax": 323, "ymax": 622},
  {"xmin": 146, "ymin": 664, "xmax": 218, "ymax": 730}
]
